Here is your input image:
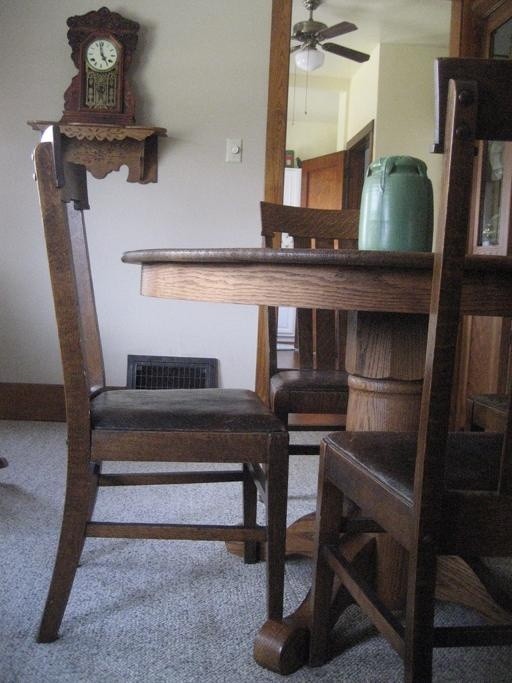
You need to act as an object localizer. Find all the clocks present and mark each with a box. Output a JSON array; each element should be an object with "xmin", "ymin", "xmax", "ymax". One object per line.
[{"xmin": 59, "ymin": 6, "xmax": 142, "ymax": 124}]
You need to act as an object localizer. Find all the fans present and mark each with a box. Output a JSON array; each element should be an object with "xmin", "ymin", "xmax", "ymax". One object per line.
[{"xmin": 288, "ymin": 0, "xmax": 372, "ymax": 65}]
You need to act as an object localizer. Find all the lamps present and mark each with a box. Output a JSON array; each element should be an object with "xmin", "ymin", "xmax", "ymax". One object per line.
[{"xmin": 293, "ymin": 48, "xmax": 325, "ymax": 74}]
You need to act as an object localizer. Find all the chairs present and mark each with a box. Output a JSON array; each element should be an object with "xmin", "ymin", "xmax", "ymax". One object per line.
[
  {"xmin": 260, "ymin": 202, "xmax": 358, "ymax": 457},
  {"xmin": 34, "ymin": 124, "xmax": 291, "ymax": 647},
  {"xmin": 306, "ymin": 56, "xmax": 510, "ymax": 683}
]
